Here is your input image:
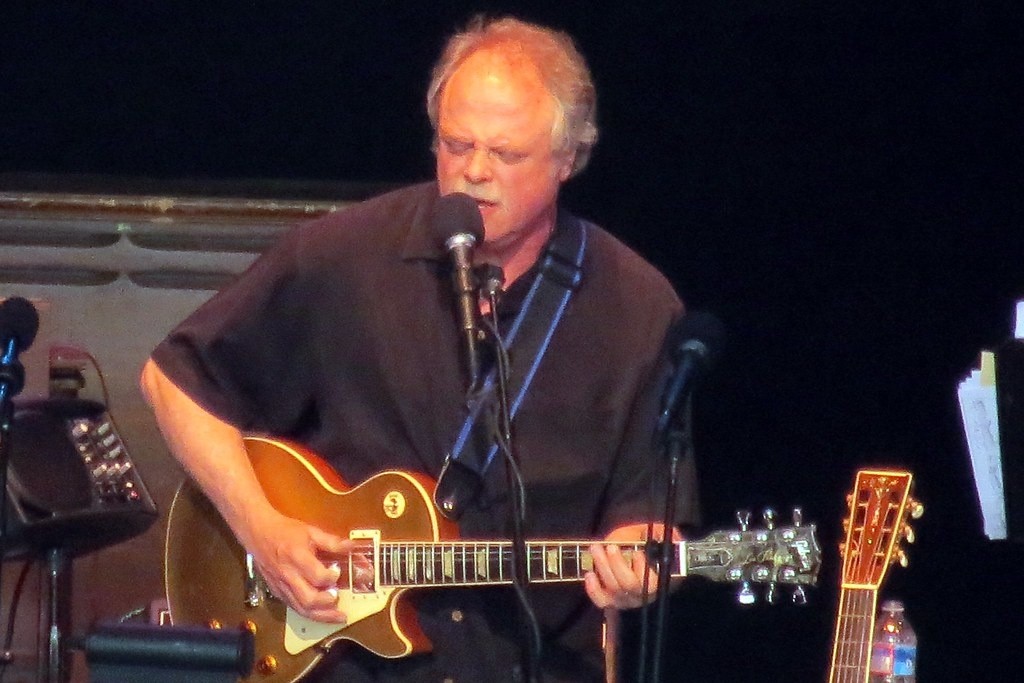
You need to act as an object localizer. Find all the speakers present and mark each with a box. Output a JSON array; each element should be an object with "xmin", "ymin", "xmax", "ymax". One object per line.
[{"xmin": 3, "ymin": 398, "xmax": 159, "ymax": 562}]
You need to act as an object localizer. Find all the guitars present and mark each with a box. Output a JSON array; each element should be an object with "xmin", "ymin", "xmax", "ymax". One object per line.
[
  {"xmin": 821, "ymin": 469, "xmax": 926, "ymax": 681},
  {"xmin": 166, "ymin": 433, "xmax": 824, "ymax": 683}
]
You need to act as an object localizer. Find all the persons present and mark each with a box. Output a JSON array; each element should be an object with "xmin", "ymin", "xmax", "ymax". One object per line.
[{"xmin": 140, "ymin": 18, "xmax": 702, "ymax": 683}]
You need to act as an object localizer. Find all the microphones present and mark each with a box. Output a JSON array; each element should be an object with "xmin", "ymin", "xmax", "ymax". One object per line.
[
  {"xmin": 0, "ymin": 297, "xmax": 40, "ymax": 423},
  {"xmin": 429, "ymin": 192, "xmax": 486, "ymax": 382},
  {"xmin": 648, "ymin": 312, "xmax": 729, "ymax": 453}
]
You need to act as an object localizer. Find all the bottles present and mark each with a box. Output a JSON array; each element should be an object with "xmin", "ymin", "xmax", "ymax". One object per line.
[{"xmin": 867, "ymin": 599, "xmax": 917, "ymax": 683}]
